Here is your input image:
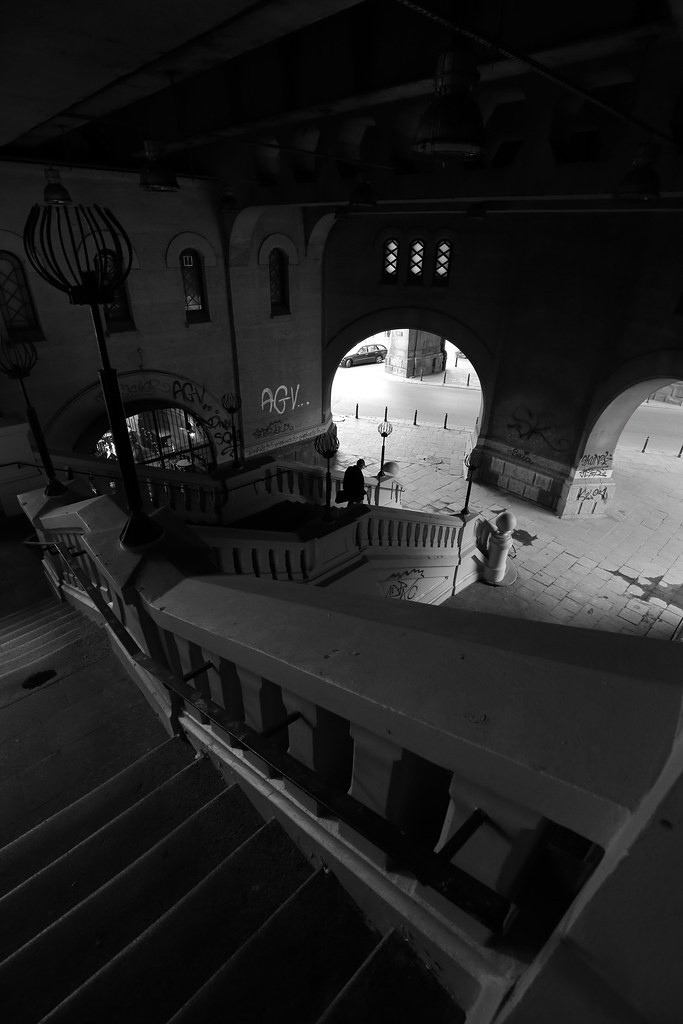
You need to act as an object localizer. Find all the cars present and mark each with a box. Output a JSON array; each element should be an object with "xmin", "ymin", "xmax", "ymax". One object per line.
[{"xmin": 339, "ymin": 344, "xmax": 388, "ymax": 367}]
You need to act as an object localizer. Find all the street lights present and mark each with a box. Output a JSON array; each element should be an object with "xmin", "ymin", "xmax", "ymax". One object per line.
[
  {"xmin": 461, "ymin": 449, "xmax": 484, "ymax": 515},
  {"xmin": 314, "ymin": 432, "xmax": 340, "ymax": 505},
  {"xmin": 376, "ymin": 421, "xmax": 393, "ymax": 477}
]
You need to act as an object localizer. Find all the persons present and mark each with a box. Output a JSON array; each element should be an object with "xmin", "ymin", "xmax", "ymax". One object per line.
[
  {"xmin": 88, "ymin": 446, "xmax": 110, "ymax": 495},
  {"xmin": 342, "ymin": 459, "xmax": 366, "ymax": 507}
]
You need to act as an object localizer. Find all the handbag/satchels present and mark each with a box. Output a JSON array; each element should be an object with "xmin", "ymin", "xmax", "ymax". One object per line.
[{"xmin": 335, "ymin": 490, "xmax": 347, "ymax": 503}]
